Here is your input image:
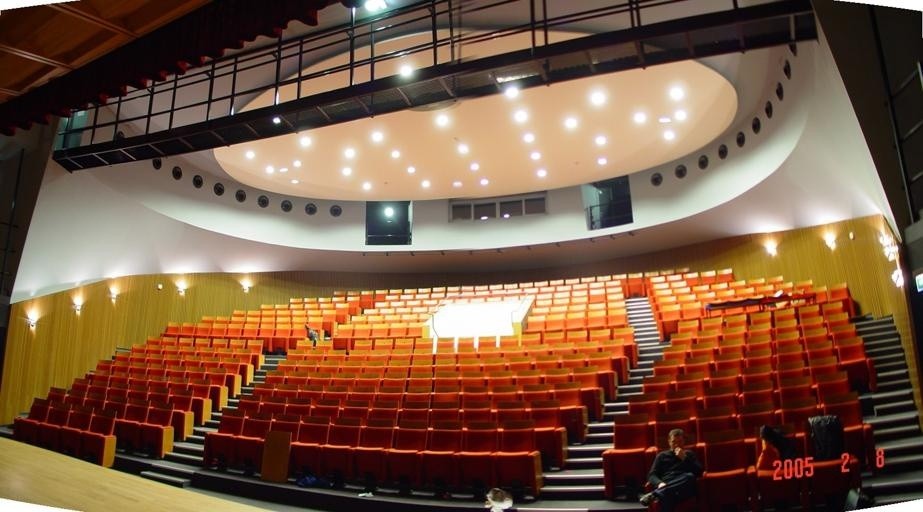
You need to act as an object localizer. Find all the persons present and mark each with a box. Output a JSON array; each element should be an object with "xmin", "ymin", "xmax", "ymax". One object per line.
[
  {"xmin": 305, "ymin": 324, "xmax": 318, "ymax": 344},
  {"xmin": 640, "ymin": 428, "xmax": 703, "ymax": 512}
]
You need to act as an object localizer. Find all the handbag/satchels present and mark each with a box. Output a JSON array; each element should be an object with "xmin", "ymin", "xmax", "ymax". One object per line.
[{"xmin": 845, "ymin": 488, "xmax": 868, "ymax": 511}]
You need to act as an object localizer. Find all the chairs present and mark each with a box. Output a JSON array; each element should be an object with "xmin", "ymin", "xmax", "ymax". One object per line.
[
  {"xmin": 11, "ymin": 321, "xmax": 201, "ymax": 469},
  {"xmin": 602, "ymin": 266, "xmax": 877, "ymax": 500},
  {"xmin": 192, "ymin": 272, "xmax": 639, "ymax": 500}
]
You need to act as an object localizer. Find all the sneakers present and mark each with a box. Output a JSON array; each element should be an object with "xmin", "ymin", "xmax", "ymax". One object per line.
[{"xmin": 640, "ymin": 493, "xmax": 656, "ymax": 506}]
